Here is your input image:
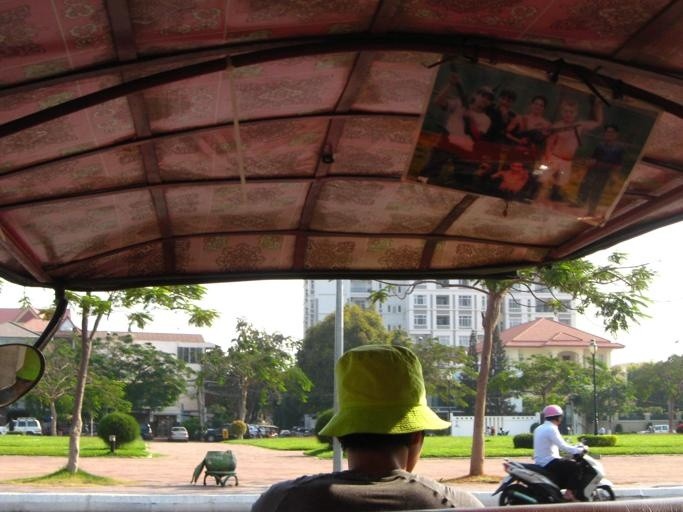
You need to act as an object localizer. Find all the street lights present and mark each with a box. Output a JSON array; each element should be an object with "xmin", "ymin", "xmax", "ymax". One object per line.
[{"xmin": 590, "ymin": 339, "xmax": 599, "ymax": 435}]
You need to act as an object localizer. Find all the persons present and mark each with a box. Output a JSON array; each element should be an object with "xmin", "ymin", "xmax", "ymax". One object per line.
[
  {"xmin": 497, "ymin": 427, "xmax": 504, "ymax": 436},
  {"xmin": 7, "ymin": 416, "xmax": 16, "ymax": 432},
  {"xmin": 250, "ymin": 342, "xmax": 485, "ymax": 512},
  {"xmin": 598, "ymin": 424, "xmax": 604, "ymax": 435},
  {"xmin": 486, "ymin": 426, "xmax": 492, "ymax": 435},
  {"xmin": 491, "ymin": 424, "xmax": 496, "ymax": 436},
  {"xmin": 605, "ymin": 418, "xmax": 612, "ymax": 434},
  {"xmin": 566, "ymin": 422, "xmax": 573, "ymax": 436},
  {"xmin": 533, "ymin": 403, "xmax": 591, "ymax": 503},
  {"xmin": 670, "ymin": 420, "xmax": 683, "ymax": 432},
  {"xmin": 410, "ymin": 62, "xmax": 634, "ymax": 219}
]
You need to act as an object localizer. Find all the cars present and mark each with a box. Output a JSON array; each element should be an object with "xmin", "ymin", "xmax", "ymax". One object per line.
[
  {"xmin": 167, "ymin": 426, "xmax": 189, "ymax": 442},
  {"xmin": 204, "ymin": 422, "xmax": 291, "ymax": 443},
  {"xmin": 636, "ymin": 424, "xmax": 677, "ymax": 434}
]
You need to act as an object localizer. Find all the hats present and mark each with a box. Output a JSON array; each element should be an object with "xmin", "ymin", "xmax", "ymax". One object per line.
[{"xmin": 316, "ymin": 344, "xmax": 453, "ymax": 439}]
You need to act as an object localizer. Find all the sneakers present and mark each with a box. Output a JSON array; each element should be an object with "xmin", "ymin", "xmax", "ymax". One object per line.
[{"xmin": 572, "ymin": 488, "xmax": 589, "ymax": 502}]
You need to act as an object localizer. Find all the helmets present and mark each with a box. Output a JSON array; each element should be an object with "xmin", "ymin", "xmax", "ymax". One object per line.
[{"xmin": 543, "ymin": 404, "xmax": 564, "ymax": 419}]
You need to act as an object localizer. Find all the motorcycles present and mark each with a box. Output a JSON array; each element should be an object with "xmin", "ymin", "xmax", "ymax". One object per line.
[
  {"xmin": 491, "ymin": 435, "xmax": 616, "ymax": 506},
  {"xmin": 142, "ymin": 427, "xmax": 154, "ymax": 440}
]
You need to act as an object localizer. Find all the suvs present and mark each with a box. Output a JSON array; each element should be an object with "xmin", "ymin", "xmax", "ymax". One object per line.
[{"xmin": 0, "ymin": 416, "xmax": 43, "ymax": 437}]
